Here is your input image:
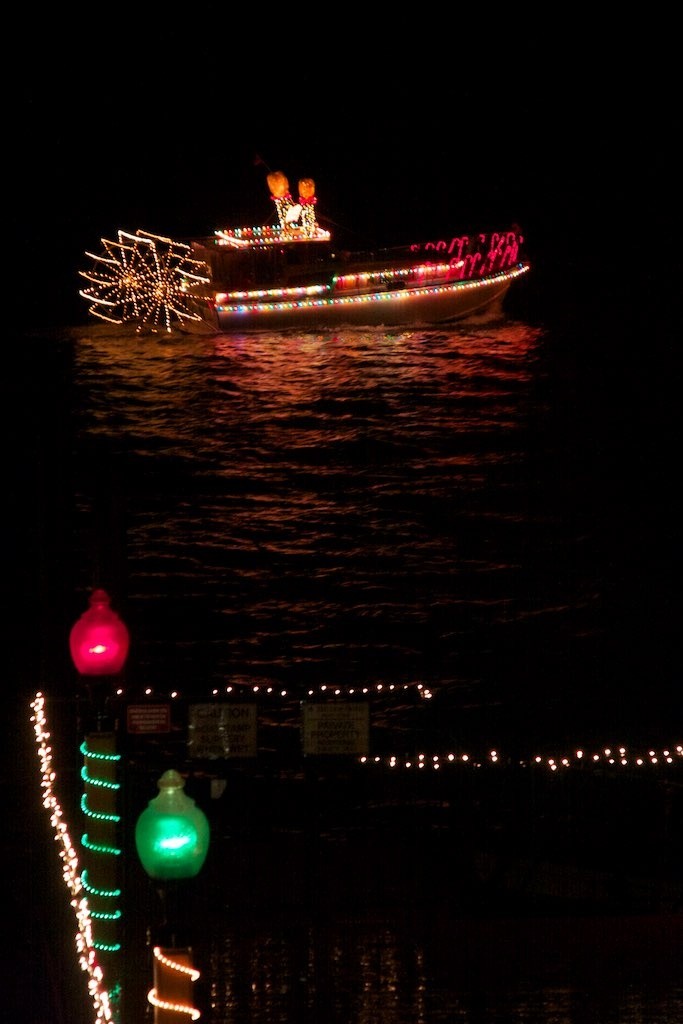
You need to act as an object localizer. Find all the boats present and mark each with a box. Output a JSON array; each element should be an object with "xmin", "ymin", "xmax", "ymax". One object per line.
[{"xmin": 77, "ymin": 169, "xmax": 535, "ymax": 337}]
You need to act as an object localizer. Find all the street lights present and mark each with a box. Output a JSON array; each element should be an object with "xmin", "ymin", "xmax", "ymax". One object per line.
[
  {"xmin": 134, "ymin": 765, "xmax": 212, "ymax": 1024},
  {"xmin": 66, "ymin": 586, "xmax": 135, "ymax": 1024}
]
